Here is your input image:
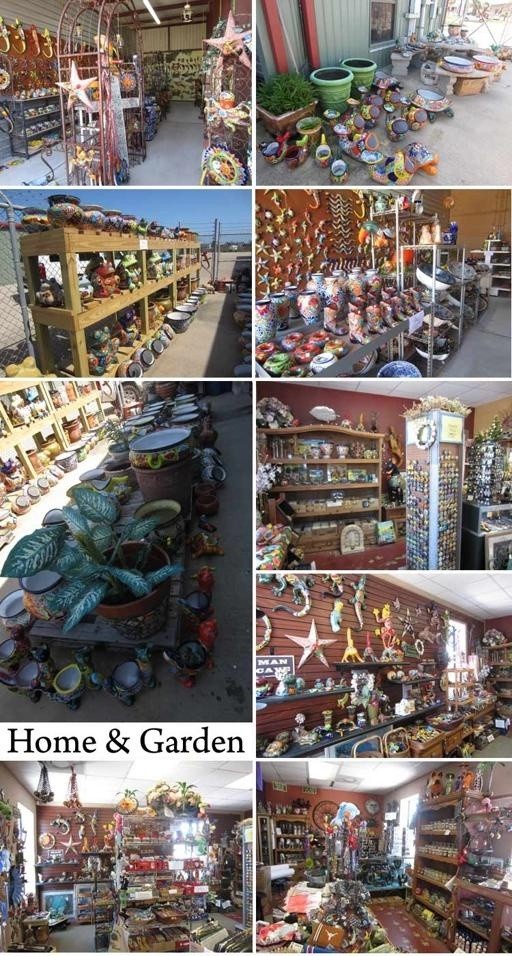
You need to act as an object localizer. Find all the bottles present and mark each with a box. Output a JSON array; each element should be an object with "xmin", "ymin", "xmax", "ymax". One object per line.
[
  {"xmin": 455, "ymin": 929, "xmax": 488, "ymax": 953},
  {"xmin": 444, "ymin": 221, "xmax": 458, "ymax": 244},
  {"xmin": 21, "ymin": 194, "xmax": 137, "ymax": 235},
  {"xmin": 256, "ymin": 268, "xmax": 422, "ymax": 379},
  {"xmin": 375, "ymin": 195, "xmax": 387, "ymax": 212}
]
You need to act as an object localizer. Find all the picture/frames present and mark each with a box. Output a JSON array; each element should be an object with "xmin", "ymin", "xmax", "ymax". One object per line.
[
  {"xmin": 40, "ymin": 890, "xmax": 76, "ymax": 923},
  {"xmin": 484, "ymin": 530, "xmax": 512, "ymax": 570}
]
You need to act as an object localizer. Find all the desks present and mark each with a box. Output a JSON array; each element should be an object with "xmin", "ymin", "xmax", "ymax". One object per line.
[{"xmin": 436, "ymin": 63, "xmax": 491, "ymax": 96}]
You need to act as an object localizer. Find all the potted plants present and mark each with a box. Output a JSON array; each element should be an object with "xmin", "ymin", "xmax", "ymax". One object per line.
[{"xmin": 257, "ymin": 68, "xmax": 320, "ymax": 136}]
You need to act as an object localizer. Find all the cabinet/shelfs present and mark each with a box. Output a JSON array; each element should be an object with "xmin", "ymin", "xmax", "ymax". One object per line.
[
  {"xmin": 478, "ymin": 642, "xmax": 512, "ymax": 716},
  {"xmin": 256, "ymin": 423, "xmax": 386, "ymax": 554},
  {"xmin": 19, "ymin": 227, "xmax": 201, "ymax": 377},
  {"xmin": 257, "ymin": 787, "xmax": 512, "ymax": 952},
  {"xmin": 82, "ymin": 813, "xmax": 210, "ymax": 952},
  {"xmin": 9, "ymin": 94, "xmax": 86, "ymax": 157},
  {"xmin": 369, "ymin": 200, "xmax": 511, "ymax": 377}
]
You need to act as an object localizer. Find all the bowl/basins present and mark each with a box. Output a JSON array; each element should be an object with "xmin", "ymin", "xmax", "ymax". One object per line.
[
  {"xmin": 309, "ymin": 442, "xmax": 349, "ymax": 460},
  {"xmin": 118, "ymin": 296, "xmax": 198, "ymax": 378},
  {"xmin": 440, "ymin": 55, "xmax": 499, "ymax": 74},
  {"xmin": 128, "ymin": 427, "xmax": 196, "ymax": 468},
  {"xmin": 415, "ymin": 261, "xmax": 489, "ymax": 360}
]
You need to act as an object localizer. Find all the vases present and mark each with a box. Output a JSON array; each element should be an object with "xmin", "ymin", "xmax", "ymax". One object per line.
[
  {"xmin": 21, "ymin": 194, "xmax": 133, "ymax": 232},
  {"xmin": 256, "ymin": 261, "xmax": 489, "ymax": 378},
  {"xmin": 117, "ymin": 287, "xmax": 207, "ymax": 377},
  {"xmin": 145, "ymin": 95, "xmax": 162, "ymax": 141},
  {"xmin": 1, "ymin": 382, "xmax": 226, "ymax": 568},
  {"xmin": 309, "ymin": 58, "xmax": 378, "ymax": 116}
]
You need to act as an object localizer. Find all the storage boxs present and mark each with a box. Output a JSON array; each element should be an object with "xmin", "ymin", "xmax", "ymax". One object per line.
[{"xmin": 408, "ymin": 723, "xmax": 464, "ymax": 758}]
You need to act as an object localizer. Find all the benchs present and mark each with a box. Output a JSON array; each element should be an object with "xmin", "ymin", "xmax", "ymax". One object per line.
[{"xmin": 389, "ymin": 40, "xmax": 466, "ymax": 76}]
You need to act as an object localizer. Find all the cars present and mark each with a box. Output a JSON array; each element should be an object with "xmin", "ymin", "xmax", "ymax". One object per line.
[{"xmin": 464, "ymin": 14, "xmax": 484, "ymax": 23}]
